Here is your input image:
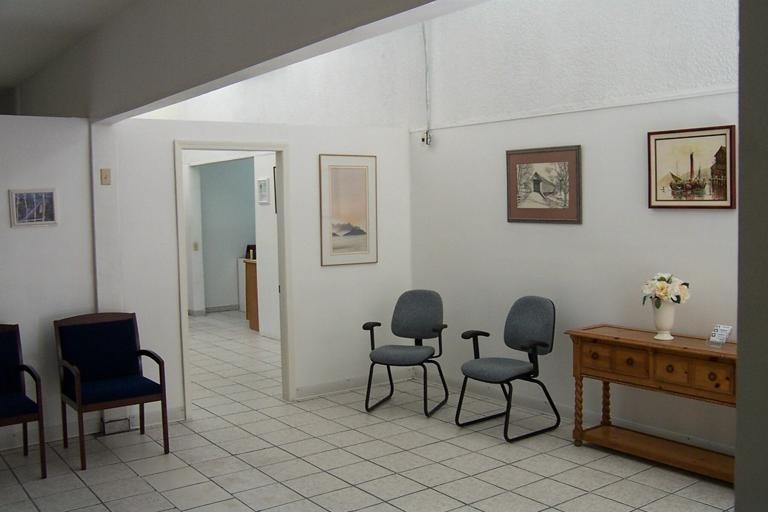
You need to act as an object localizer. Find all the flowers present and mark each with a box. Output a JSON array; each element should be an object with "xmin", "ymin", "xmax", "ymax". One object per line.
[{"xmin": 640, "ymin": 273, "xmax": 691, "ymax": 307}]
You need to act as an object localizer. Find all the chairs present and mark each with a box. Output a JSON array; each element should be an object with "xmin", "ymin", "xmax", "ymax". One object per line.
[
  {"xmin": 0, "ymin": 323, "xmax": 49, "ymax": 480},
  {"xmin": 53, "ymin": 312, "xmax": 167, "ymax": 472},
  {"xmin": 452, "ymin": 296, "xmax": 559, "ymax": 443},
  {"xmin": 359, "ymin": 289, "xmax": 450, "ymax": 419}
]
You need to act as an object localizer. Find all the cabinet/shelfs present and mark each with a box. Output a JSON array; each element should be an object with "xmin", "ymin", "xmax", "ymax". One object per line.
[{"xmin": 563, "ymin": 323, "xmax": 736, "ymax": 490}]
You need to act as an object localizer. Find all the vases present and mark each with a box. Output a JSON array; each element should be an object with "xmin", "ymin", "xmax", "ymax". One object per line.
[{"xmin": 649, "ymin": 298, "xmax": 677, "ymax": 341}]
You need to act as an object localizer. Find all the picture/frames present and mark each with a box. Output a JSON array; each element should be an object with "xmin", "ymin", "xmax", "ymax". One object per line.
[
  {"xmin": 318, "ymin": 153, "xmax": 378, "ymax": 267},
  {"xmin": 7, "ymin": 185, "xmax": 56, "ymax": 227},
  {"xmin": 647, "ymin": 124, "xmax": 736, "ymax": 210},
  {"xmin": 256, "ymin": 178, "xmax": 271, "ymax": 205},
  {"xmin": 505, "ymin": 143, "xmax": 583, "ymax": 226}
]
